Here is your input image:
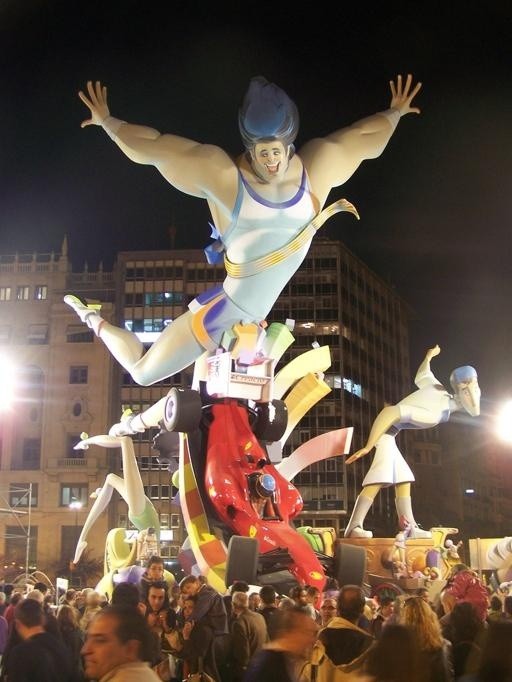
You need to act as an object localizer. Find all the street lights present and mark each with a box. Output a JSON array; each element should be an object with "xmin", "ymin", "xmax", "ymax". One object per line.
[{"xmin": 68, "ymin": 496, "xmax": 84, "ymax": 553}]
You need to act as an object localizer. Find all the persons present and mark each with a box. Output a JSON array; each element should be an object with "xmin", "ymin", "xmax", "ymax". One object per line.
[
  {"xmin": 0, "ymin": 555, "xmax": 512, "ymax": 681},
  {"xmin": 62, "ymin": 73, "xmax": 422, "ymax": 386},
  {"xmin": 340, "ymin": 344, "xmax": 481, "ymax": 539},
  {"xmin": 71, "ymin": 434, "xmax": 161, "ymax": 566}
]
